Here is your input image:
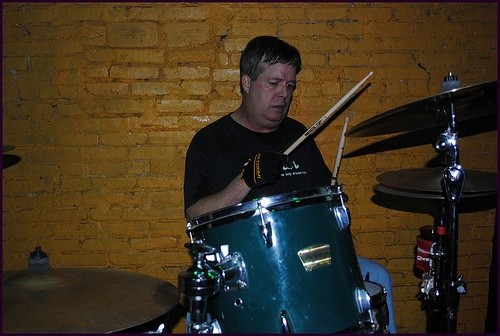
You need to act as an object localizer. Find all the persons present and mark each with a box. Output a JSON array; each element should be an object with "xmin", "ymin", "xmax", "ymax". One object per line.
[{"xmin": 182, "ymin": 36, "xmax": 396, "ymax": 334}]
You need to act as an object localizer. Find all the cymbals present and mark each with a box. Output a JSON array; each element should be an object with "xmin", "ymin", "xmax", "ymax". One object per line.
[
  {"xmin": 345, "ymin": 80, "xmax": 500, "ymax": 138},
  {"xmin": 373, "ymin": 166, "xmax": 499, "ymax": 202},
  {"xmin": 1, "ymin": 270, "xmax": 180, "ymax": 336}
]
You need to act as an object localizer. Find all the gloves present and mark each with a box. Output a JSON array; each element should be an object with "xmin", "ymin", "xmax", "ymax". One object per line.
[{"xmin": 243, "ymin": 150, "xmax": 291, "ymax": 187}]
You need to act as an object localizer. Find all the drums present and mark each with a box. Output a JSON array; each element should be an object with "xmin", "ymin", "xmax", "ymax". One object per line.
[
  {"xmin": 186, "ymin": 186, "xmax": 380, "ymax": 335},
  {"xmin": 363, "ymin": 280, "xmax": 391, "ymax": 335}
]
